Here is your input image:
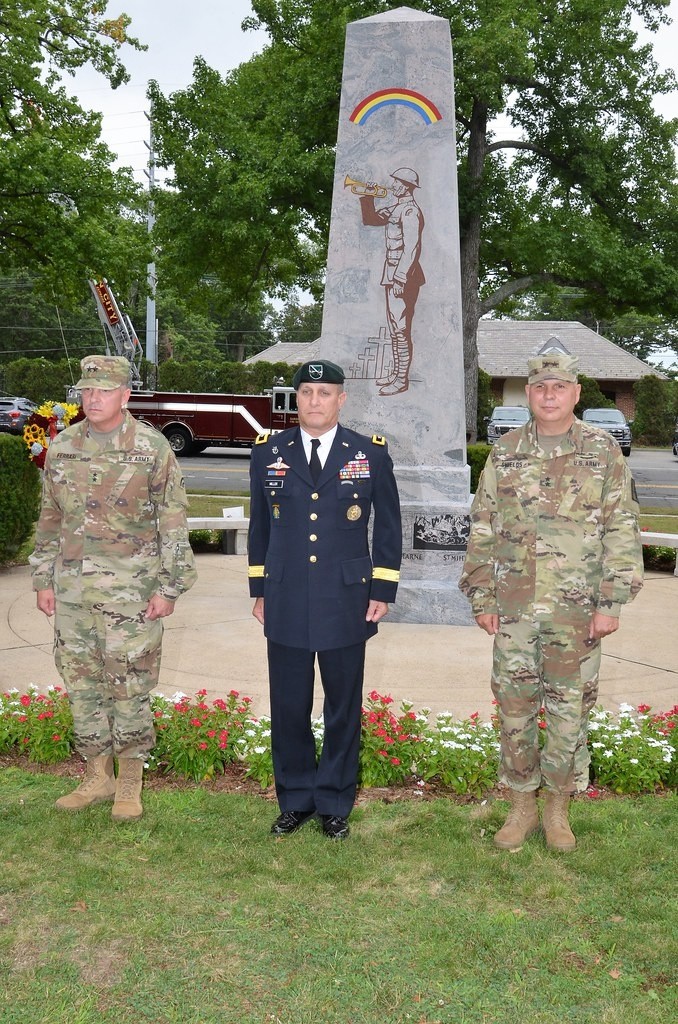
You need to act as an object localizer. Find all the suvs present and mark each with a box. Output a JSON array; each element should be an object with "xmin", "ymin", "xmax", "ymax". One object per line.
[
  {"xmin": 582, "ymin": 408, "xmax": 634, "ymax": 457},
  {"xmin": 0, "ymin": 394, "xmax": 42, "ymax": 437},
  {"xmin": 482, "ymin": 406, "xmax": 531, "ymax": 445}
]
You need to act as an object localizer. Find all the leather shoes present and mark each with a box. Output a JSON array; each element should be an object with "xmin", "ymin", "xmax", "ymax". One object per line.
[
  {"xmin": 270, "ymin": 806, "xmax": 316, "ymax": 835},
  {"xmin": 323, "ymin": 814, "xmax": 349, "ymax": 838}
]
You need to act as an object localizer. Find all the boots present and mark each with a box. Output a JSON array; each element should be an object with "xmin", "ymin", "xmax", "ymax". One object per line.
[
  {"xmin": 494, "ymin": 789, "xmax": 539, "ymax": 850},
  {"xmin": 542, "ymin": 791, "xmax": 576, "ymax": 851},
  {"xmin": 56, "ymin": 755, "xmax": 117, "ymax": 809},
  {"xmin": 113, "ymin": 758, "xmax": 144, "ymax": 817}
]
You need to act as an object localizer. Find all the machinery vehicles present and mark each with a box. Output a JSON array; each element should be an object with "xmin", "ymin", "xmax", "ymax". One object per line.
[{"xmin": 66, "ymin": 276, "xmax": 300, "ymax": 456}]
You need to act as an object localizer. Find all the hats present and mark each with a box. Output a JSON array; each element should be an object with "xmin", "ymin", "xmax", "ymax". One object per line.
[
  {"xmin": 74, "ymin": 355, "xmax": 131, "ymax": 390},
  {"xmin": 527, "ymin": 356, "xmax": 578, "ymax": 386},
  {"xmin": 292, "ymin": 360, "xmax": 346, "ymax": 391}
]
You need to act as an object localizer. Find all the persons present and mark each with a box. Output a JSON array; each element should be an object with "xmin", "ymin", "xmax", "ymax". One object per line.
[
  {"xmin": 247, "ymin": 358, "xmax": 404, "ymax": 842},
  {"xmin": 458, "ymin": 355, "xmax": 646, "ymax": 855},
  {"xmin": 28, "ymin": 354, "xmax": 198, "ymax": 819}
]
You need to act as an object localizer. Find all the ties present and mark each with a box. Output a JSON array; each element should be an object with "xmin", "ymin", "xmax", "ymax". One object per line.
[{"xmin": 308, "ymin": 439, "xmax": 322, "ymax": 486}]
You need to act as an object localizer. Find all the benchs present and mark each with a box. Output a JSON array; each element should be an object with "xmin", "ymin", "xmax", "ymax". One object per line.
[
  {"xmin": 186, "ymin": 517, "xmax": 250, "ymax": 556},
  {"xmin": 640, "ymin": 532, "xmax": 678, "ymax": 576}
]
